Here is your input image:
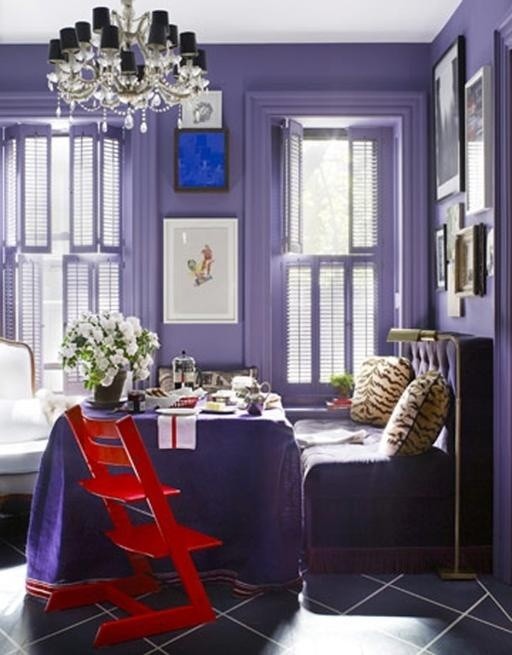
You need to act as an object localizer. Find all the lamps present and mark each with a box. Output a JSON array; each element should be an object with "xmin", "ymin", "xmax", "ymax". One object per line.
[{"xmin": 45, "ymin": 0, "xmax": 210, "ymax": 133}]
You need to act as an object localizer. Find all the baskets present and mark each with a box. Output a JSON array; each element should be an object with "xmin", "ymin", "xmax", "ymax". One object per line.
[{"xmin": 155, "ymin": 398, "xmax": 198, "ymax": 408}]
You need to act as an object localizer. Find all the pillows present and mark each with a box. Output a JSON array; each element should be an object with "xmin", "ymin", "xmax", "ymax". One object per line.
[
  {"xmin": 382, "ymin": 370, "xmax": 454, "ymax": 456},
  {"xmin": 0, "ymin": 399, "xmax": 50, "ymax": 444},
  {"xmin": 159, "ymin": 367, "xmax": 259, "ymax": 393},
  {"xmin": 350, "ymin": 356, "xmax": 413, "ymax": 427}
]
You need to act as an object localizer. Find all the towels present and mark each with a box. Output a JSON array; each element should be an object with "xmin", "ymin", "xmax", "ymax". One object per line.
[{"xmin": 158, "ymin": 413, "xmax": 199, "ymax": 450}]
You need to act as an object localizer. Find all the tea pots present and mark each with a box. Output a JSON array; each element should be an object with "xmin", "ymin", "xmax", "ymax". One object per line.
[{"xmin": 243, "ymin": 382, "xmax": 271, "ymax": 415}]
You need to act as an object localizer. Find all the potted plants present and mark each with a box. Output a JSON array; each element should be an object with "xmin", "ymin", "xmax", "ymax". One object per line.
[{"xmin": 325, "ymin": 372, "xmax": 355, "ymax": 409}]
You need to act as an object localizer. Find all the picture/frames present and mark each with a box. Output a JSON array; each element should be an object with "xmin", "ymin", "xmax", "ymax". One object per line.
[
  {"xmin": 182, "ymin": 91, "xmax": 222, "ymax": 128},
  {"xmin": 163, "ymin": 217, "xmax": 239, "ymax": 325},
  {"xmin": 174, "ymin": 128, "xmax": 230, "ymax": 193},
  {"xmin": 432, "ymin": 34, "xmax": 492, "ymax": 318}
]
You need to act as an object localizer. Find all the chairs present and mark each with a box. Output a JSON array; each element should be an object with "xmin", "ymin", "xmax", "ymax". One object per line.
[{"xmin": 0, "ymin": 336, "xmax": 48, "ymax": 509}]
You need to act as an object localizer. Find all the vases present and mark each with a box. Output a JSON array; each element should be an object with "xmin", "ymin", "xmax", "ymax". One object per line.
[{"xmin": 93, "ymin": 369, "xmax": 127, "ymax": 401}]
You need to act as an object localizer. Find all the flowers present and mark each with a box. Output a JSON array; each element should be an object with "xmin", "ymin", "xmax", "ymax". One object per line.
[{"xmin": 60, "ymin": 310, "xmax": 161, "ymax": 390}]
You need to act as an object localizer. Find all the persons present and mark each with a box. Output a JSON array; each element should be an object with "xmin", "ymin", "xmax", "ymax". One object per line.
[{"xmin": 199, "ymin": 244, "xmax": 215, "ymax": 277}]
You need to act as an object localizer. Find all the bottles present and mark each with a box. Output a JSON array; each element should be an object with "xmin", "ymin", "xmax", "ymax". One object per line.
[{"xmin": 172, "ymin": 349, "xmax": 195, "ymax": 389}]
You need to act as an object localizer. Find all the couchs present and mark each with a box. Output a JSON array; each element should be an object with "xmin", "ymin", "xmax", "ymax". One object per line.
[{"xmin": 295, "ymin": 332, "xmax": 494, "ymax": 574}]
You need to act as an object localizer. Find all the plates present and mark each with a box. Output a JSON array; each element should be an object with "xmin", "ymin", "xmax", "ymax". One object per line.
[
  {"xmin": 155, "ymin": 408, "xmax": 200, "ymax": 416},
  {"xmin": 200, "ymin": 404, "xmax": 236, "ymax": 413}
]
